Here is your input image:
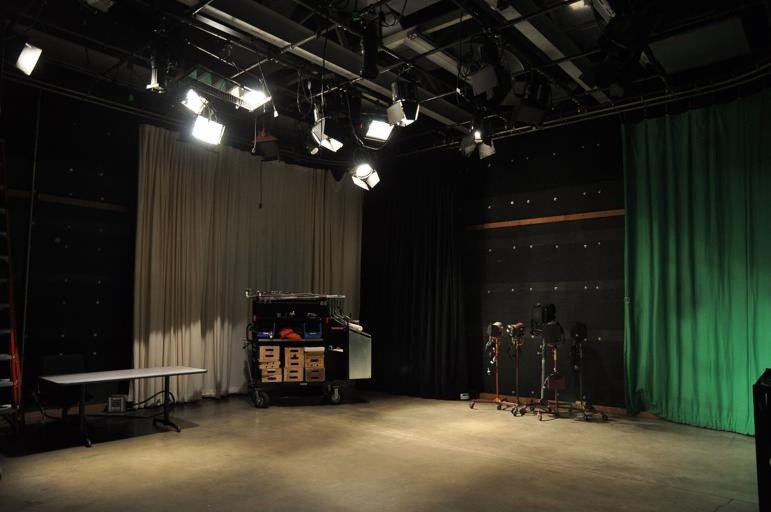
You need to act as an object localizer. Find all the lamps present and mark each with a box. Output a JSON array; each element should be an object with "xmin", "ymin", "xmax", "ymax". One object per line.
[
  {"xmin": 303, "ymin": 32, "xmax": 421, "ymax": 192},
  {"xmin": 470, "ymin": 300, "xmax": 609, "ymax": 423},
  {"xmin": 250, "ymin": 111, "xmax": 280, "ymax": 164}
]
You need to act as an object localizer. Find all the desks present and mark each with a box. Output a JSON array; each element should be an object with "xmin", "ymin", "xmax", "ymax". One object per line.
[{"xmin": 38, "ymin": 365, "xmax": 208, "ymax": 448}]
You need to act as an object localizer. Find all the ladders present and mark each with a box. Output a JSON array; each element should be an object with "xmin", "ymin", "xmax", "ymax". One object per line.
[{"xmin": 0, "ymin": 139, "xmax": 27, "ymax": 436}]
